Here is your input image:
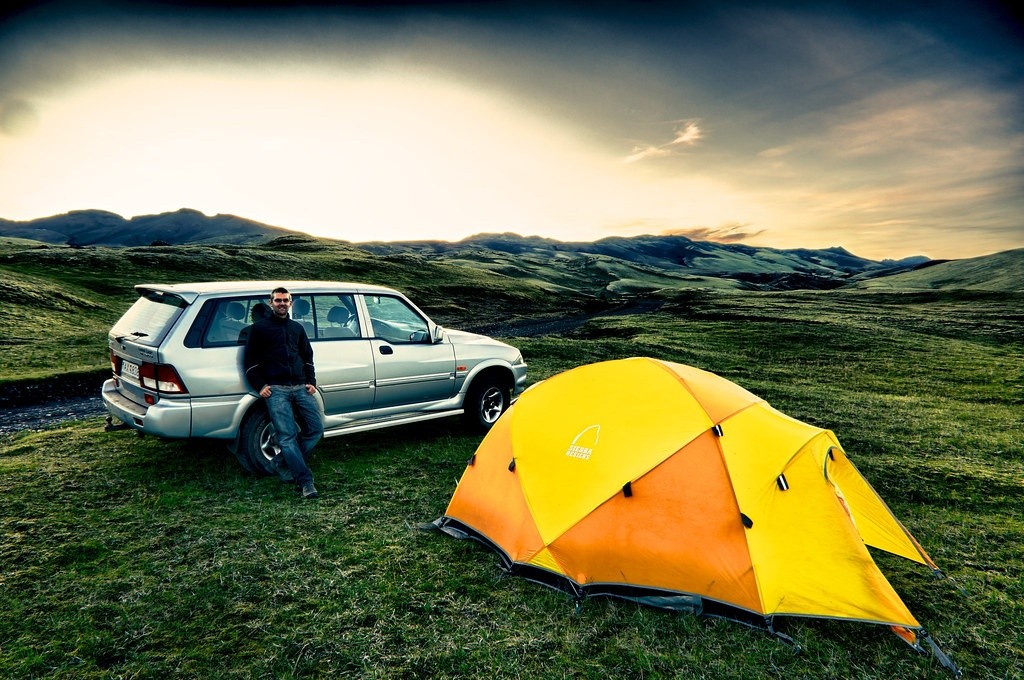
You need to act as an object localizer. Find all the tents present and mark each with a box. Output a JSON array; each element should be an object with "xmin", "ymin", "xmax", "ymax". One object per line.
[{"xmin": 441, "ymin": 355, "xmax": 938, "ymax": 652}]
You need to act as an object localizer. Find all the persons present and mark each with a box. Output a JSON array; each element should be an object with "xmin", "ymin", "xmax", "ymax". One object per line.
[{"xmin": 243, "ymin": 287, "xmax": 324, "ymax": 497}]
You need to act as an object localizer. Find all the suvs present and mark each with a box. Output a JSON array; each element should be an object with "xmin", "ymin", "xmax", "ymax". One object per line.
[{"xmin": 101, "ymin": 279, "xmax": 529, "ymax": 479}]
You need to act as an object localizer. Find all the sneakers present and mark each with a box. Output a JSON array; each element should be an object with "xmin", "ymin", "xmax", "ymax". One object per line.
[
  {"xmin": 271, "ymin": 455, "xmax": 294, "ymax": 483},
  {"xmin": 302, "ymin": 481, "xmax": 318, "ymax": 498}
]
